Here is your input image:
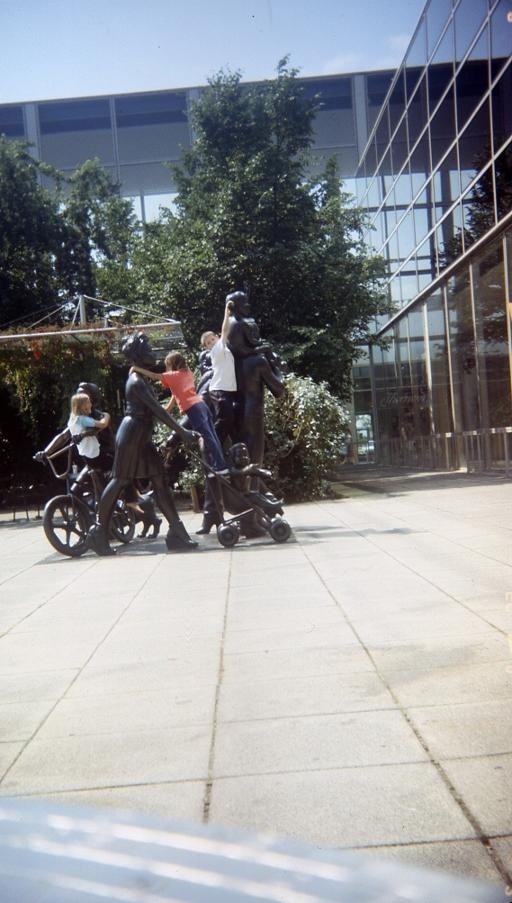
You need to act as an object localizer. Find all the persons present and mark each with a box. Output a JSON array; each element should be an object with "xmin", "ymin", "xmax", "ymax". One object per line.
[
  {"xmin": 129, "ymin": 351, "xmax": 228, "ymax": 472},
  {"xmin": 84, "ymin": 331, "xmax": 199, "ymax": 559},
  {"xmin": 227, "ymin": 292, "xmax": 280, "ymax": 376},
  {"xmin": 33, "ymin": 383, "xmax": 116, "ymax": 545},
  {"xmin": 234, "ymin": 318, "xmax": 288, "ymax": 539},
  {"xmin": 227, "ymin": 442, "xmax": 284, "ymax": 511},
  {"xmin": 161, "ymin": 352, "xmax": 225, "ymax": 535},
  {"xmin": 68, "ymin": 393, "xmax": 144, "ymax": 513},
  {"xmin": 201, "ymin": 302, "xmax": 236, "ymax": 464}
]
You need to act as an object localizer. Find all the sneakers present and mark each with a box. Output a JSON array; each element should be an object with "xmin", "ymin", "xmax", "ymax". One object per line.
[
  {"xmin": 204, "ymin": 468, "xmax": 229, "ymax": 478},
  {"xmin": 126, "ymin": 502, "xmax": 144, "ymax": 514}
]
